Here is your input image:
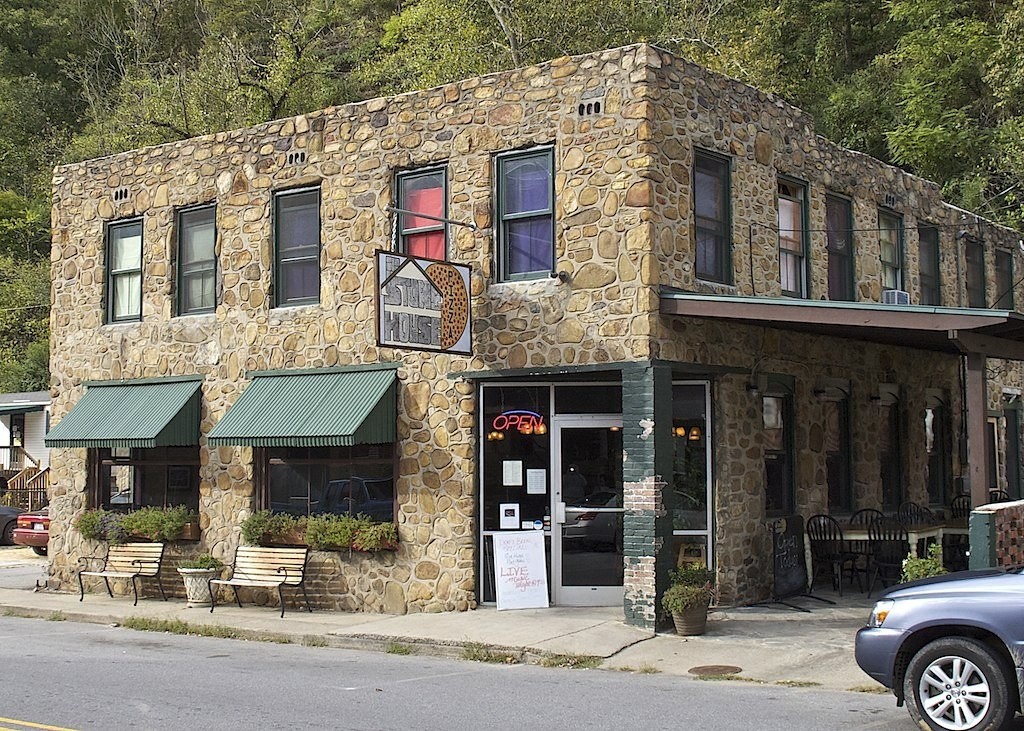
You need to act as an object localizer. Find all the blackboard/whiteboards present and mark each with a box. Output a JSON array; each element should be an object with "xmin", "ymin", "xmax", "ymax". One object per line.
[
  {"xmin": 493, "ymin": 530, "xmax": 549, "ymax": 611},
  {"xmin": 772, "ymin": 515, "xmax": 808, "ymax": 597}
]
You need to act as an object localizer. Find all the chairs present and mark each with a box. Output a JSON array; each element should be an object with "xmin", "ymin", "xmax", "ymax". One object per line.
[{"xmin": 808, "ymin": 490, "xmax": 1010, "ymax": 601}]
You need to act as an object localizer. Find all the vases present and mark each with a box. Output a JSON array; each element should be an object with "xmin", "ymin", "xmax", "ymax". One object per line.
[
  {"xmin": 257, "ymin": 533, "xmax": 397, "ymax": 551},
  {"xmin": 97, "ymin": 523, "xmax": 199, "ymax": 542}
]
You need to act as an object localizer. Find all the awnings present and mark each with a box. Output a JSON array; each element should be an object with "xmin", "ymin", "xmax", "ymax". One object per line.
[
  {"xmin": 43, "ymin": 380, "xmax": 201, "ymax": 450},
  {"xmin": 206, "ymin": 369, "xmax": 395, "ymax": 448},
  {"xmin": 0, "ymin": 405, "xmax": 44, "ymax": 416}
]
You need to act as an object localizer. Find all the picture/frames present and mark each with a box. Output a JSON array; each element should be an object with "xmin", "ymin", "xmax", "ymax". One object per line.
[{"xmin": 166, "ymin": 465, "xmax": 192, "ymax": 491}]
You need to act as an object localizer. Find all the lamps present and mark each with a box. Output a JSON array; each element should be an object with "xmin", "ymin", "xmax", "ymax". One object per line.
[
  {"xmin": 676, "ymin": 427, "xmax": 701, "ymax": 440},
  {"xmin": 487, "ymin": 431, "xmax": 505, "ymax": 442},
  {"xmin": 519, "ymin": 388, "xmax": 549, "ymax": 435}
]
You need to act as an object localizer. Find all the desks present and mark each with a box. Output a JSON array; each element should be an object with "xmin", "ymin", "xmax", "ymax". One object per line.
[
  {"xmin": 803, "ymin": 522, "xmax": 945, "ymax": 594},
  {"xmin": 942, "ymin": 517, "xmax": 969, "ymax": 535}
]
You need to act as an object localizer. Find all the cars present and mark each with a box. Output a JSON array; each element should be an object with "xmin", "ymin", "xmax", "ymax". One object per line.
[
  {"xmin": 12, "ymin": 505, "xmax": 49, "ymax": 556},
  {"xmin": 110, "ymin": 488, "xmax": 130, "ymax": 503},
  {"xmin": 0, "ymin": 506, "xmax": 28, "ymax": 546},
  {"xmin": 854, "ymin": 560, "xmax": 1024, "ymax": 731},
  {"xmin": 557, "ymin": 485, "xmax": 708, "ymax": 555}
]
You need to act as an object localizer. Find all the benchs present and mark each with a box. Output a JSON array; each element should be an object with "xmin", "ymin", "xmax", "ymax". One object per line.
[
  {"xmin": 207, "ymin": 549, "xmax": 312, "ymax": 618},
  {"xmin": 77, "ymin": 543, "xmax": 167, "ymax": 606}
]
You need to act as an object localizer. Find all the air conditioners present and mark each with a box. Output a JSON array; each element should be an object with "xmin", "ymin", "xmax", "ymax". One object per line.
[{"xmin": 882, "ymin": 288, "xmax": 911, "ymax": 305}]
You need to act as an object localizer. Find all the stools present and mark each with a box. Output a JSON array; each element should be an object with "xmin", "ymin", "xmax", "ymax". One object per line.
[{"xmin": 677, "ymin": 543, "xmax": 706, "ymax": 569}]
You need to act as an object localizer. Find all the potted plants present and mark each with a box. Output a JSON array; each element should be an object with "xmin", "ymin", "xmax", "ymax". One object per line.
[
  {"xmin": 176, "ymin": 554, "xmax": 225, "ymax": 608},
  {"xmin": 659, "ymin": 583, "xmax": 712, "ymax": 639}
]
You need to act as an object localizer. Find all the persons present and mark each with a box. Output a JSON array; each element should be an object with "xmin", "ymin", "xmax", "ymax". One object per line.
[{"xmin": 562, "ymin": 464, "xmax": 587, "ymax": 504}]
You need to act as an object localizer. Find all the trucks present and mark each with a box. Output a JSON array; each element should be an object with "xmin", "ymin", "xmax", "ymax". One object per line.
[{"xmin": 267, "ymin": 476, "xmax": 393, "ymax": 525}]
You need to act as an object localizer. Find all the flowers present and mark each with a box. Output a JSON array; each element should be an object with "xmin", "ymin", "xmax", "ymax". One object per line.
[
  {"xmin": 239, "ymin": 509, "xmax": 399, "ymax": 553},
  {"xmin": 70, "ymin": 504, "xmax": 197, "ymax": 550}
]
